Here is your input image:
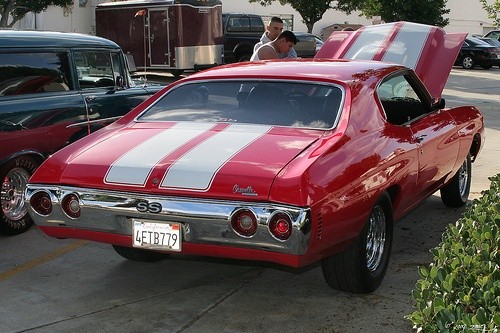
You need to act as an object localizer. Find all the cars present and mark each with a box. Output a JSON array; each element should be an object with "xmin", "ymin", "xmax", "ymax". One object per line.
[
  {"xmin": 478, "ymin": 37, "xmax": 500, "ymax": 67},
  {"xmin": 24, "ymin": 21, "xmax": 486, "ymax": 295},
  {"xmin": 315, "ymin": 37, "xmax": 324, "ymax": 54},
  {"xmin": 452, "ymin": 36, "xmax": 497, "ymax": 68},
  {"xmin": 281, "ymin": 31, "xmax": 316, "ymax": 58},
  {"xmin": 0, "ymin": 29, "xmax": 176, "ymax": 234},
  {"xmin": 481, "ymin": 30, "xmax": 500, "ymax": 41}
]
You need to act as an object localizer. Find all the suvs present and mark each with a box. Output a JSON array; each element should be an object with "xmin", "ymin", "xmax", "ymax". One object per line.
[{"xmin": 222, "ymin": 14, "xmax": 266, "ymax": 63}]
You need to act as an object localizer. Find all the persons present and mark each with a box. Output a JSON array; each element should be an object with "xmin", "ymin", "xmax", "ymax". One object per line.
[
  {"xmin": 249, "ymin": 30, "xmax": 300, "ymax": 61},
  {"xmin": 253, "ymin": 16, "xmax": 297, "ymax": 59}
]
